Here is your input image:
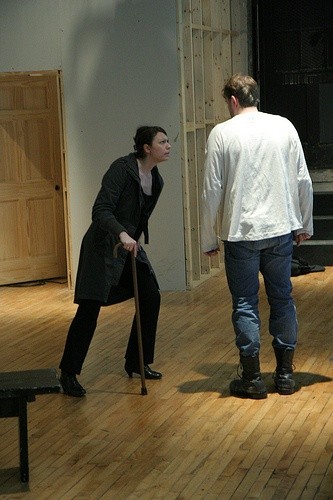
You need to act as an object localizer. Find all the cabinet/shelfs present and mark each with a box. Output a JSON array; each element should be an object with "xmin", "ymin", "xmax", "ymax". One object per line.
[{"xmin": 59, "ymin": 0, "xmax": 250, "ymax": 293}]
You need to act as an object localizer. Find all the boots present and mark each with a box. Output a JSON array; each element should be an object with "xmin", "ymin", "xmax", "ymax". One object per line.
[
  {"xmin": 230, "ymin": 353, "xmax": 267, "ymax": 399},
  {"xmin": 272, "ymin": 350, "xmax": 299, "ymax": 395}
]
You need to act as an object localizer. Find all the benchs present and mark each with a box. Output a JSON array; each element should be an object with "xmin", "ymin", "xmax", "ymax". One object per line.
[{"xmin": 0, "ymin": 367, "xmax": 64, "ymax": 483}]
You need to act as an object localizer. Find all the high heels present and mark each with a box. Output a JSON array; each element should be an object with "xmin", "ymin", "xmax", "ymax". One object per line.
[
  {"xmin": 124, "ymin": 361, "xmax": 162, "ymax": 379},
  {"xmin": 60, "ymin": 375, "xmax": 87, "ymax": 397}
]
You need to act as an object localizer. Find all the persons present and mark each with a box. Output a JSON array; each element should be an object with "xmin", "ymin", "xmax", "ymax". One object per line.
[
  {"xmin": 58, "ymin": 125, "xmax": 172, "ymax": 398},
  {"xmin": 200, "ymin": 73, "xmax": 314, "ymax": 399}
]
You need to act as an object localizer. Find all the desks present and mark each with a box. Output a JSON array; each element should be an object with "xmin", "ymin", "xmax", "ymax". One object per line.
[{"xmin": 292, "ymin": 168, "xmax": 333, "ymax": 246}]
